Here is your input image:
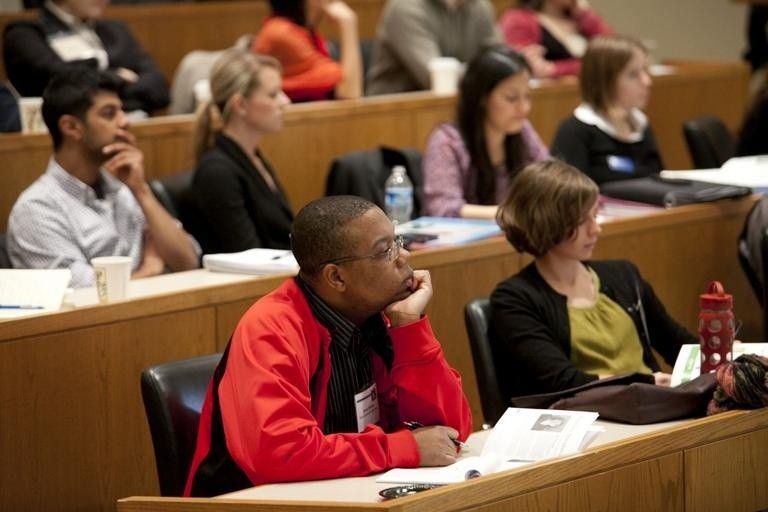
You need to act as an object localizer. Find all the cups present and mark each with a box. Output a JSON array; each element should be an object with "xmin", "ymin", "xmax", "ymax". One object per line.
[
  {"xmin": 88, "ymin": 255, "xmax": 134, "ymax": 305},
  {"xmin": 695, "ymin": 279, "xmax": 737, "ymax": 375}
]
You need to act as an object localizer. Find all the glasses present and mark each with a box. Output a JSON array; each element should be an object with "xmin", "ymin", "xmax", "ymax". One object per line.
[{"xmin": 322, "ymin": 235, "xmax": 406, "ymax": 266}]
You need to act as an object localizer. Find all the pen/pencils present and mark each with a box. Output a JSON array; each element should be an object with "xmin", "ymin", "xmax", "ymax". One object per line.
[
  {"xmin": 0, "ymin": 304, "xmax": 45, "ymax": 311},
  {"xmin": 403, "ymin": 419, "xmax": 468, "ymax": 449}
]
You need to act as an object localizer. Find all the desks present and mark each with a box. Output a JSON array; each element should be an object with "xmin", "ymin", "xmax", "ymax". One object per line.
[
  {"xmin": 1, "ymin": 58, "xmax": 751, "ymax": 232},
  {"xmin": 116, "ymin": 406, "xmax": 767, "ymax": 512},
  {"xmin": 1, "ymin": 167, "xmax": 768, "ymax": 512},
  {"xmin": 4, "ymin": 2, "xmax": 519, "ymax": 82}
]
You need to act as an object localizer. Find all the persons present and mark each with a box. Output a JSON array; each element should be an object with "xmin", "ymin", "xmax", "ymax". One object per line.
[
  {"xmin": 423, "ymin": 49, "xmax": 551, "ymax": 218},
  {"xmin": 554, "ymin": 35, "xmax": 664, "ymax": 185},
  {"xmin": 188, "ymin": 50, "xmax": 293, "ymax": 252},
  {"xmin": 368, "ymin": 0, "xmax": 553, "ymax": 93},
  {"xmin": 488, "ymin": 160, "xmax": 700, "ymax": 391},
  {"xmin": 248, "ymin": 0, "xmax": 361, "ymax": 102},
  {"xmin": 184, "ymin": 197, "xmax": 473, "ymax": 498},
  {"xmin": 6, "ymin": 0, "xmax": 169, "ymax": 120},
  {"xmin": 7, "ymin": 68, "xmax": 199, "ymax": 287},
  {"xmin": 500, "ymin": 0, "xmax": 618, "ymax": 78}
]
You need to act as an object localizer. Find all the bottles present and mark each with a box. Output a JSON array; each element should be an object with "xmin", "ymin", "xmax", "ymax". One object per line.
[
  {"xmin": 384, "ymin": 165, "xmax": 413, "ymax": 227},
  {"xmin": 699, "ymin": 282, "xmax": 733, "ymax": 374}
]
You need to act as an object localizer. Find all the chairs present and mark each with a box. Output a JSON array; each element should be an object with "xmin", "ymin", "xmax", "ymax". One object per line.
[
  {"xmin": 326, "ymin": 147, "xmax": 424, "ymax": 221},
  {"xmin": 140, "ymin": 352, "xmax": 254, "ymax": 497},
  {"xmin": 736, "ymin": 193, "xmax": 768, "ymax": 315},
  {"xmin": 462, "ymin": 295, "xmax": 512, "ymax": 427},
  {"xmin": 147, "ymin": 172, "xmax": 198, "ymax": 224},
  {"xmin": 329, "ymin": 37, "xmax": 378, "ymax": 79},
  {"xmin": 682, "ymin": 116, "xmax": 735, "ymax": 168}
]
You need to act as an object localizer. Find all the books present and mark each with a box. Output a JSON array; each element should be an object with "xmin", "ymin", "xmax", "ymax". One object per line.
[
  {"xmin": 376, "ymin": 456, "xmax": 489, "ymax": 485},
  {"xmin": 479, "ymin": 407, "xmax": 602, "ymax": 469},
  {"xmin": 669, "ymin": 342, "xmax": 768, "ymax": 388},
  {"xmin": 660, "ymin": 156, "xmax": 768, "ymax": 187},
  {"xmin": 203, "ymin": 248, "xmax": 300, "ymax": 276},
  {"xmin": 0, "ymin": 268, "xmax": 71, "ymax": 317}
]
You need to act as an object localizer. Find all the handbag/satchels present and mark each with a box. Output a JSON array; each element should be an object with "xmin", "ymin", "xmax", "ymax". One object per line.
[
  {"xmin": 511, "ymin": 372, "xmax": 720, "ymax": 425},
  {"xmin": 600, "ymin": 174, "xmax": 752, "ymax": 208}
]
